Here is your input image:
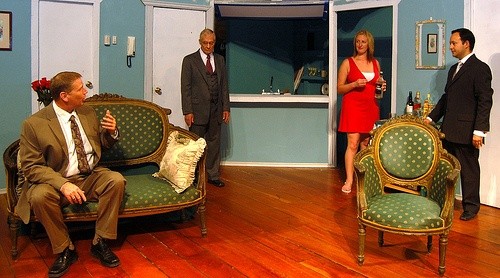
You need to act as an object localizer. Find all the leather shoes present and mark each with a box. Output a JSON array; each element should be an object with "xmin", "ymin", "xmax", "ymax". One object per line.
[
  {"xmin": 460, "ymin": 210, "xmax": 475, "ymax": 220},
  {"xmin": 90, "ymin": 238, "xmax": 120, "ymax": 268},
  {"xmin": 207, "ymin": 178, "xmax": 224, "ymax": 187},
  {"xmin": 48, "ymin": 245, "xmax": 78, "ymax": 277}
]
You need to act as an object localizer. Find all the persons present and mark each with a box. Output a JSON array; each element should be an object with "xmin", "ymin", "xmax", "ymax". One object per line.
[
  {"xmin": 181, "ymin": 28, "xmax": 230, "ymax": 187},
  {"xmin": 337, "ymin": 29, "xmax": 386, "ymax": 194},
  {"xmin": 428, "ymin": 28, "xmax": 494, "ymax": 220},
  {"xmin": 16, "ymin": 72, "xmax": 127, "ymax": 278}
]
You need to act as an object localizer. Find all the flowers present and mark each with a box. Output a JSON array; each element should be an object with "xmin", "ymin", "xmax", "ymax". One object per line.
[{"xmin": 31, "ymin": 78, "xmax": 53, "ymax": 107}]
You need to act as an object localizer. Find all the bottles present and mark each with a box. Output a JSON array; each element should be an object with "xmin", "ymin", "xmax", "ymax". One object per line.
[
  {"xmin": 424, "ymin": 93, "xmax": 432, "ymax": 119},
  {"xmin": 414, "ymin": 91, "xmax": 422, "ymax": 115},
  {"xmin": 406, "ymin": 91, "xmax": 414, "ymax": 116},
  {"xmin": 321, "ymin": 64, "xmax": 326, "ymax": 78},
  {"xmin": 375, "ymin": 72, "xmax": 384, "ymax": 98}
]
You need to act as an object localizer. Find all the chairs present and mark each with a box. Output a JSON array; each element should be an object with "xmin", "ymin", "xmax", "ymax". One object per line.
[{"xmin": 353, "ymin": 114, "xmax": 462, "ymax": 278}]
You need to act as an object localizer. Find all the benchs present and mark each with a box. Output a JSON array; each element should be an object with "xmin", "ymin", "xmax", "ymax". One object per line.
[{"xmin": 3, "ymin": 93, "xmax": 208, "ymax": 264}]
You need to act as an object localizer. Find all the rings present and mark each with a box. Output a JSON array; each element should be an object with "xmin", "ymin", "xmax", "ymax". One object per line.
[{"xmin": 72, "ymin": 197, "xmax": 76, "ymax": 200}]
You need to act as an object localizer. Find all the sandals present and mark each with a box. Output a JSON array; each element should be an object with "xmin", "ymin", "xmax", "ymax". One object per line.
[{"xmin": 342, "ymin": 180, "xmax": 351, "ymax": 193}]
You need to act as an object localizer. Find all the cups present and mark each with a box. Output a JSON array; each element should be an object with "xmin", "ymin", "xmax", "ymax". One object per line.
[
  {"xmin": 417, "ymin": 108, "xmax": 424, "ymax": 118},
  {"xmin": 428, "ymin": 102, "xmax": 435, "ymax": 114}
]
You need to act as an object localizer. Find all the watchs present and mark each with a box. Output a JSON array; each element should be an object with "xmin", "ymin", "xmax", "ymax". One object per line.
[{"xmin": 110, "ymin": 129, "xmax": 117, "ymax": 137}]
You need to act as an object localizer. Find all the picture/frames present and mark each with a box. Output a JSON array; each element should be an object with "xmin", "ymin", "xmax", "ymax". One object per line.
[
  {"xmin": 0, "ymin": 11, "xmax": 12, "ymax": 51},
  {"xmin": 427, "ymin": 34, "xmax": 437, "ymax": 53}
]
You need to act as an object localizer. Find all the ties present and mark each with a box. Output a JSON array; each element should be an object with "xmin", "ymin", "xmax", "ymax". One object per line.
[
  {"xmin": 70, "ymin": 114, "xmax": 91, "ymax": 174},
  {"xmin": 456, "ymin": 61, "xmax": 462, "ymax": 74},
  {"xmin": 206, "ymin": 54, "xmax": 213, "ymax": 74}
]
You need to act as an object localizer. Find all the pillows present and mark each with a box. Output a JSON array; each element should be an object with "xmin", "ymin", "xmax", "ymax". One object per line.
[
  {"xmin": 15, "ymin": 148, "xmax": 27, "ymax": 199},
  {"xmin": 152, "ymin": 130, "xmax": 207, "ymax": 193}
]
